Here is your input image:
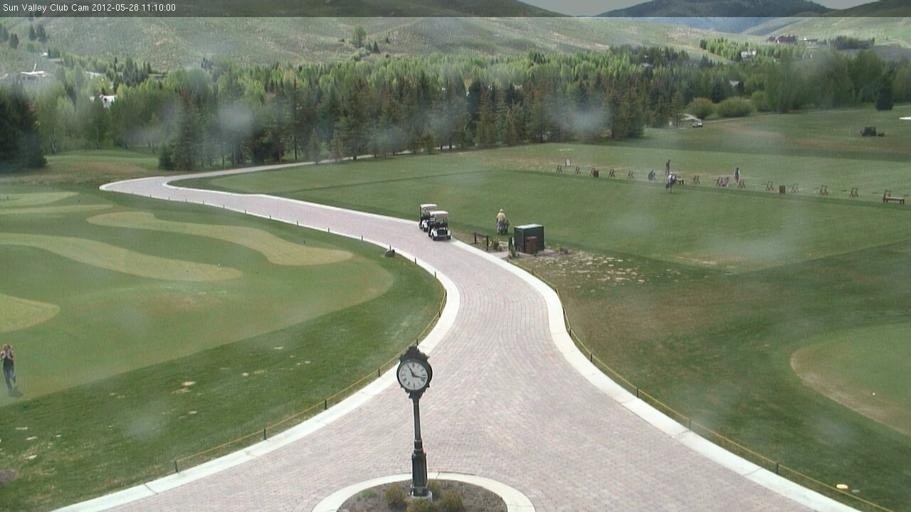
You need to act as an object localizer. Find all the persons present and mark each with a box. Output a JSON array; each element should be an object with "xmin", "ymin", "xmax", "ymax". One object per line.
[
  {"xmin": 496, "ymin": 208, "xmax": 506, "ymax": 235},
  {"xmin": 667, "ymin": 172, "xmax": 674, "ymax": 193},
  {"xmin": 0, "ymin": 344, "xmax": 18, "ymax": 394},
  {"xmin": 734, "ymin": 168, "xmax": 739, "ymax": 184}
]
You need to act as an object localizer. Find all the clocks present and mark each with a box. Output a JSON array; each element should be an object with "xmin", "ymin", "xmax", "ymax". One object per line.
[{"xmin": 397, "ymin": 359, "xmax": 432, "ymax": 392}]
